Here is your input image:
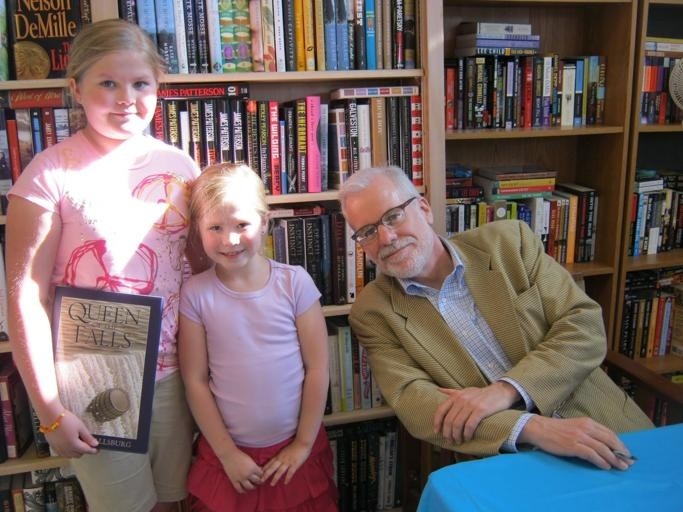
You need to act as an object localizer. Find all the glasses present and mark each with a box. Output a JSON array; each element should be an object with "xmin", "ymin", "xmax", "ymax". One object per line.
[{"xmin": 352, "ymin": 196, "xmax": 417, "ymax": 245}]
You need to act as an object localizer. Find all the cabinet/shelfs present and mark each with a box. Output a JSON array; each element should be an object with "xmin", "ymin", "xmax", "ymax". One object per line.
[
  {"xmin": 0, "ymin": 0, "xmax": 445, "ymax": 512},
  {"xmin": 614, "ymin": 1, "xmax": 683, "ymax": 375},
  {"xmin": 444, "ymin": 0, "xmax": 641, "ymax": 366}
]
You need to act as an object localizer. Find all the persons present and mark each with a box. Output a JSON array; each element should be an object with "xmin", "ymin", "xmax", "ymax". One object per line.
[
  {"xmin": 1, "ymin": 17, "xmax": 209, "ymax": 511},
  {"xmin": 175, "ymin": 159, "xmax": 338, "ymax": 511},
  {"xmin": 335, "ymin": 163, "xmax": 658, "ymax": 472}
]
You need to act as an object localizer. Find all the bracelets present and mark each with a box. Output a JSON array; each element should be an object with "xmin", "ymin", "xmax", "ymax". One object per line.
[{"xmin": 34, "ymin": 407, "xmax": 68, "ymax": 434}]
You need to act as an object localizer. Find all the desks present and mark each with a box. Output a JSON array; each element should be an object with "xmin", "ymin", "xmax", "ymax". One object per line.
[{"xmin": 414, "ymin": 421, "xmax": 682, "ymax": 512}]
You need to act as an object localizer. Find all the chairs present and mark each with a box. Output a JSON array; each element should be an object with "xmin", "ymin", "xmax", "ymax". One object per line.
[{"xmin": 420, "ymin": 350, "xmax": 682, "ymax": 498}]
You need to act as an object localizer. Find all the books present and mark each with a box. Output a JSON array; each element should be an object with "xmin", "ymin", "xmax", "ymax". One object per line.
[
  {"xmin": 0, "ymin": 84, "xmax": 421, "ymax": 216},
  {"xmin": 0, "ymin": 469, "xmax": 86, "ymax": 512},
  {"xmin": 0, "ymin": 1, "xmax": 425, "ymax": 82},
  {"xmin": 444, "ymin": 163, "xmax": 600, "ymax": 267},
  {"xmin": 626, "ymin": 167, "xmax": 682, "ymax": 256},
  {"xmin": 638, "ymin": 36, "xmax": 682, "ymax": 125},
  {"xmin": 49, "ymin": 282, "xmax": 164, "ymax": 455},
  {"xmin": 0, "ymin": 242, "xmax": 10, "ymax": 341},
  {"xmin": 318, "ymin": 416, "xmax": 447, "ymax": 512},
  {"xmin": 442, "ymin": 21, "xmax": 608, "ymax": 133},
  {"xmin": 0, "ymin": 366, "xmax": 32, "ymax": 460},
  {"xmin": 616, "ymin": 268, "xmax": 681, "ymax": 427},
  {"xmin": 323, "ymin": 314, "xmax": 390, "ymax": 415},
  {"xmin": 28, "ymin": 401, "xmax": 51, "ymax": 459},
  {"xmin": 263, "ymin": 201, "xmax": 382, "ymax": 305}
]
